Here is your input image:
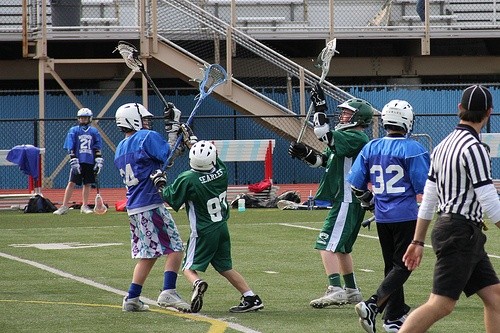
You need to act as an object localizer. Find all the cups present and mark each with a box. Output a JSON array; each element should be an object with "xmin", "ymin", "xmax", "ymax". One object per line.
[{"xmin": 238, "ymin": 198, "xmax": 245, "ymax": 211}]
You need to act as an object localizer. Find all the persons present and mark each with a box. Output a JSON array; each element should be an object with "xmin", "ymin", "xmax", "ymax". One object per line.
[
  {"xmin": 53, "ymin": 108, "xmax": 104, "ymax": 216},
  {"xmin": 114, "ymin": 103, "xmax": 191, "ymax": 314},
  {"xmin": 288, "ymin": 83, "xmax": 374, "ymax": 309},
  {"xmin": 345, "ymin": 100, "xmax": 430, "ymax": 333},
  {"xmin": 149, "ymin": 140, "xmax": 264, "ymax": 314},
  {"xmin": 397, "ymin": 88, "xmax": 500, "ymax": 333},
  {"xmin": 416, "ymin": 0, "xmax": 425, "ymax": 25}
]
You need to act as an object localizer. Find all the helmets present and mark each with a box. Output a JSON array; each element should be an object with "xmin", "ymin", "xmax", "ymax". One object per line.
[
  {"xmin": 188, "ymin": 140, "xmax": 216, "ymax": 171},
  {"xmin": 76, "ymin": 108, "xmax": 93, "ymax": 123},
  {"xmin": 334, "ymin": 98, "xmax": 374, "ymax": 131},
  {"xmin": 381, "ymin": 100, "xmax": 414, "ymax": 134},
  {"xmin": 115, "ymin": 103, "xmax": 154, "ymax": 132}
]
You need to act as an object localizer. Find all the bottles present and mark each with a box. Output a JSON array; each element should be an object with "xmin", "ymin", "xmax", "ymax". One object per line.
[{"xmin": 307, "ymin": 196, "xmax": 314, "ymax": 211}]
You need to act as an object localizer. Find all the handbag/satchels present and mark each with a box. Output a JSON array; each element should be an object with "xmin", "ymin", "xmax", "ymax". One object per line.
[{"xmin": 25, "ymin": 195, "xmax": 57, "ymax": 214}]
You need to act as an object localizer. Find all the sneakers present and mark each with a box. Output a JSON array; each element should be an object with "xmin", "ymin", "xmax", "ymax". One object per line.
[
  {"xmin": 340, "ymin": 285, "xmax": 363, "ymax": 304},
  {"xmin": 383, "ymin": 314, "xmax": 409, "ymax": 333},
  {"xmin": 309, "ymin": 285, "xmax": 349, "ymax": 308},
  {"xmin": 229, "ymin": 294, "xmax": 264, "ymax": 313},
  {"xmin": 157, "ymin": 288, "xmax": 190, "ymax": 312},
  {"xmin": 355, "ymin": 295, "xmax": 380, "ymax": 333},
  {"xmin": 190, "ymin": 280, "xmax": 208, "ymax": 313},
  {"xmin": 121, "ymin": 294, "xmax": 150, "ymax": 312}
]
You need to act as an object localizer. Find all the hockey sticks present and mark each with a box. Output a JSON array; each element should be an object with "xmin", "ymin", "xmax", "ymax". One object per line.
[
  {"xmin": 93, "ymin": 177, "xmax": 107, "ymax": 215},
  {"xmin": 161, "ymin": 64, "xmax": 228, "ymax": 176},
  {"xmin": 113, "ymin": 40, "xmax": 169, "ymax": 108},
  {"xmin": 289, "ymin": 39, "xmax": 339, "ymax": 161}
]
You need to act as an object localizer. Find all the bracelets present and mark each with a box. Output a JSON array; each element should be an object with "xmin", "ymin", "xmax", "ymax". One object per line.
[{"xmin": 411, "ymin": 240, "xmax": 424, "ymax": 247}]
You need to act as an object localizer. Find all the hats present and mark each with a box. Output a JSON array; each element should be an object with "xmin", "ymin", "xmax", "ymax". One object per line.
[{"xmin": 460, "ymin": 83, "xmax": 494, "ymax": 111}]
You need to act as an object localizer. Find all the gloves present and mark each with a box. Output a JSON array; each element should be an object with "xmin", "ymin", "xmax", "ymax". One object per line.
[
  {"xmin": 149, "ymin": 168, "xmax": 167, "ymax": 189},
  {"xmin": 178, "ymin": 121, "xmax": 197, "ymax": 145},
  {"xmin": 308, "ymin": 81, "xmax": 329, "ymax": 112},
  {"xmin": 93, "ymin": 157, "xmax": 104, "ymax": 175},
  {"xmin": 163, "ymin": 102, "xmax": 182, "ymax": 134},
  {"xmin": 69, "ymin": 158, "xmax": 80, "ymax": 175},
  {"xmin": 288, "ymin": 142, "xmax": 310, "ymax": 160}
]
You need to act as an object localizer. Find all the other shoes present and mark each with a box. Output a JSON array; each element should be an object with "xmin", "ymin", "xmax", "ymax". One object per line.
[
  {"xmin": 80, "ymin": 205, "xmax": 93, "ymax": 214},
  {"xmin": 53, "ymin": 205, "xmax": 68, "ymax": 215}
]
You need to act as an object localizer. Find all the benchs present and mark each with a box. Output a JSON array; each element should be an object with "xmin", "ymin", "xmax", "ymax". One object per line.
[
  {"xmin": 402, "ymin": 15, "xmax": 457, "ymax": 30},
  {"xmin": 238, "ymin": 17, "xmax": 286, "ymax": 32},
  {"xmin": 80, "ymin": 18, "xmax": 119, "ymax": 31}
]
